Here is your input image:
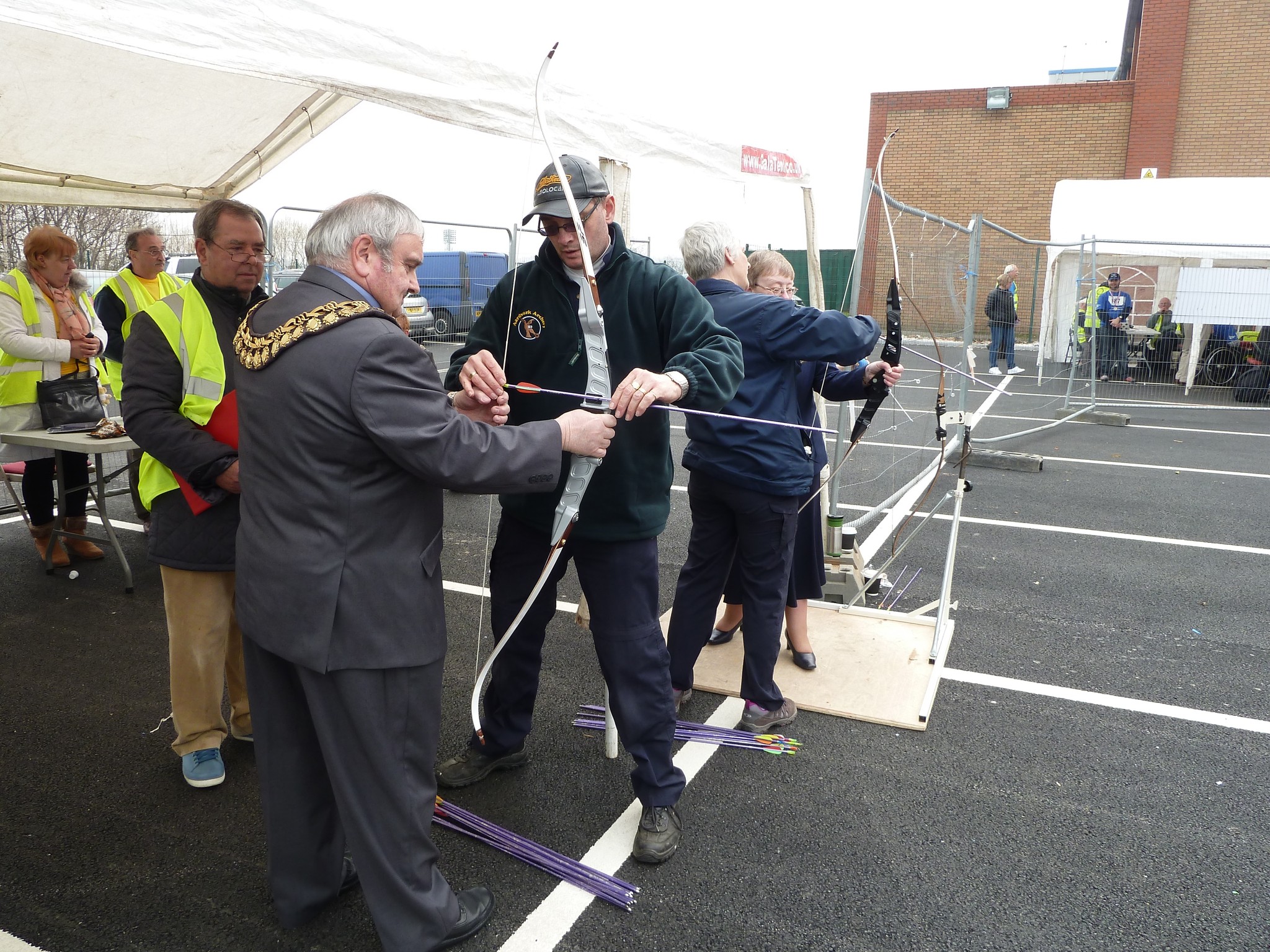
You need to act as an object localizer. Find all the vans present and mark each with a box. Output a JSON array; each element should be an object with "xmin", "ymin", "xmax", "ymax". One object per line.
[{"xmin": 414, "ymin": 251, "xmax": 510, "ymax": 341}]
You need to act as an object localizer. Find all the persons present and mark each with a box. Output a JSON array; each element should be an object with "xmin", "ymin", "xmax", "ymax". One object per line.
[
  {"xmin": 985, "ymin": 265, "xmax": 1025, "ymax": 376},
  {"xmin": 120, "ymin": 200, "xmax": 273, "ymax": 789},
  {"xmin": 234, "ymin": 193, "xmax": 617, "ymax": 952},
  {"xmin": 434, "ymin": 154, "xmax": 744, "ymax": 862},
  {"xmin": 706, "ymin": 250, "xmax": 904, "ymax": 669},
  {"xmin": 1072, "ymin": 273, "xmax": 1185, "ymax": 383},
  {"xmin": 666, "ymin": 220, "xmax": 881, "ymax": 734},
  {"xmin": 395, "ymin": 313, "xmax": 437, "ymax": 369},
  {"xmin": 0, "ymin": 228, "xmax": 108, "ymax": 568},
  {"xmin": 92, "ymin": 227, "xmax": 185, "ymax": 535}
]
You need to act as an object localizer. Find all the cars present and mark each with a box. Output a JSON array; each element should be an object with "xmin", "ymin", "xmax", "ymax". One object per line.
[
  {"xmin": 401, "ymin": 293, "xmax": 436, "ymax": 344},
  {"xmin": 270, "ymin": 268, "xmax": 305, "ymax": 296}
]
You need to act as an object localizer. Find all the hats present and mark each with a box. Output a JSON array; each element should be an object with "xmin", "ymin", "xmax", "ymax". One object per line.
[
  {"xmin": 1108, "ymin": 273, "xmax": 1120, "ymax": 280},
  {"xmin": 522, "ymin": 154, "xmax": 610, "ymax": 226}
]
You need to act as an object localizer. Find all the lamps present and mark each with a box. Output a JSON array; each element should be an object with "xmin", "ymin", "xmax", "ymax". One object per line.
[{"xmin": 986, "ymin": 86, "xmax": 1012, "ymax": 110}]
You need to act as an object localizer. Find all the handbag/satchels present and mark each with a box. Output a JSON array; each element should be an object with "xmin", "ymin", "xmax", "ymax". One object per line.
[{"xmin": 36, "ymin": 332, "xmax": 106, "ymax": 429}]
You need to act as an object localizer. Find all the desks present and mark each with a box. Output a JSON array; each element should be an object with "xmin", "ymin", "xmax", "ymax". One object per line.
[
  {"xmin": 0, "ymin": 415, "xmax": 140, "ymax": 594},
  {"xmin": 1109, "ymin": 325, "xmax": 1161, "ymax": 378}
]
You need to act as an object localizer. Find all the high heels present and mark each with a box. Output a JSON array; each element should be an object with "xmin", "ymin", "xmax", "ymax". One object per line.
[
  {"xmin": 784, "ymin": 627, "xmax": 817, "ymax": 669},
  {"xmin": 708, "ymin": 617, "xmax": 743, "ymax": 645}
]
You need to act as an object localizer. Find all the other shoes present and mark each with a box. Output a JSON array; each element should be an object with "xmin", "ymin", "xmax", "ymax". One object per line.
[
  {"xmin": 989, "ymin": 367, "xmax": 1002, "ymax": 374},
  {"xmin": 1174, "ymin": 379, "xmax": 1186, "ymax": 386},
  {"xmin": 1120, "ymin": 377, "xmax": 1136, "ymax": 382},
  {"xmin": 1007, "ymin": 366, "xmax": 1025, "ymax": 374},
  {"xmin": 997, "ymin": 352, "xmax": 1007, "ymax": 359},
  {"xmin": 1100, "ymin": 375, "xmax": 1108, "ymax": 381}
]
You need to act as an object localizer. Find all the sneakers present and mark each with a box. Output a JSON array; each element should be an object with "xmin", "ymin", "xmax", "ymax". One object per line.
[
  {"xmin": 435, "ymin": 739, "xmax": 528, "ymax": 788},
  {"xmin": 741, "ymin": 697, "xmax": 797, "ymax": 735},
  {"xmin": 182, "ymin": 747, "xmax": 225, "ymax": 787},
  {"xmin": 672, "ymin": 687, "xmax": 692, "ymax": 718},
  {"xmin": 233, "ymin": 734, "xmax": 254, "ymax": 742},
  {"xmin": 633, "ymin": 800, "xmax": 683, "ymax": 863}
]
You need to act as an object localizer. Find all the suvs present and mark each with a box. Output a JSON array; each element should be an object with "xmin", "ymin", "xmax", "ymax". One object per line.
[{"xmin": 118, "ymin": 252, "xmax": 201, "ymax": 283}]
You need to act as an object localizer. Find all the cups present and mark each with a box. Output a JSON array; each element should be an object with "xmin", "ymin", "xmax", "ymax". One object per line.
[
  {"xmin": 842, "ymin": 526, "xmax": 857, "ymax": 551},
  {"xmin": 864, "ymin": 570, "xmax": 883, "ymax": 596},
  {"xmin": 69, "ymin": 570, "xmax": 79, "ymax": 579}
]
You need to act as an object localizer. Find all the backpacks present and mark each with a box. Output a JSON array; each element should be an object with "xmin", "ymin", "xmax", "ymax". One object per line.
[{"xmin": 1233, "ymin": 366, "xmax": 1269, "ymax": 403}]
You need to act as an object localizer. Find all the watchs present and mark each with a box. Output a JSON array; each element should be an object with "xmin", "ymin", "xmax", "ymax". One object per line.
[{"xmin": 664, "ymin": 371, "xmax": 689, "ymax": 401}]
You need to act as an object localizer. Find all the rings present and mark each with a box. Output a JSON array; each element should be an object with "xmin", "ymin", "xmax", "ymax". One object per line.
[
  {"xmin": 639, "ymin": 388, "xmax": 647, "ymax": 395},
  {"xmin": 630, "ymin": 382, "xmax": 641, "ymax": 390},
  {"xmin": 469, "ymin": 372, "xmax": 478, "ymax": 381},
  {"xmin": 649, "ymin": 391, "xmax": 658, "ymax": 400}
]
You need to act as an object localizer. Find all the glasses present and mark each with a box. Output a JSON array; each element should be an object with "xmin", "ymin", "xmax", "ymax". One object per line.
[
  {"xmin": 204, "ymin": 238, "xmax": 273, "ymax": 263},
  {"xmin": 537, "ymin": 197, "xmax": 602, "ymax": 236},
  {"xmin": 133, "ymin": 249, "xmax": 170, "ymax": 258},
  {"xmin": 755, "ymin": 284, "xmax": 799, "ymax": 297}
]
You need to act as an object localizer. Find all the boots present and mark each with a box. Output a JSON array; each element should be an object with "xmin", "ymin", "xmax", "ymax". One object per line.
[
  {"xmin": 27, "ymin": 517, "xmax": 71, "ymax": 567},
  {"xmin": 56, "ymin": 515, "xmax": 105, "ymax": 559}
]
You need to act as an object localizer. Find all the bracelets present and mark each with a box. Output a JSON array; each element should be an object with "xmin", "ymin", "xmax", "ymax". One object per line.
[
  {"xmin": 862, "ymin": 376, "xmax": 868, "ymax": 385},
  {"xmin": 448, "ymin": 391, "xmax": 458, "ymax": 407}
]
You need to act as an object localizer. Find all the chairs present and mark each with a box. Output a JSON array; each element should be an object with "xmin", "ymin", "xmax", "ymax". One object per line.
[
  {"xmin": 0, "ymin": 462, "xmax": 118, "ymax": 537},
  {"xmin": 1064, "ymin": 328, "xmax": 1083, "ymax": 371}
]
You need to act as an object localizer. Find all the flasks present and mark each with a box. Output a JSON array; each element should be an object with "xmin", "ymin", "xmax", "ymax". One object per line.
[{"xmin": 826, "ymin": 513, "xmax": 844, "ymax": 556}]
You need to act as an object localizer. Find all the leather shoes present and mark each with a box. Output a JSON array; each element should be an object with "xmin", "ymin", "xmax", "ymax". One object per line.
[
  {"xmin": 339, "ymin": 850, "xmax": 360, "ymax": 893},
  {"xmin": 431, "ymin": 885, "xmax": 495, "ymax": 950}
]
